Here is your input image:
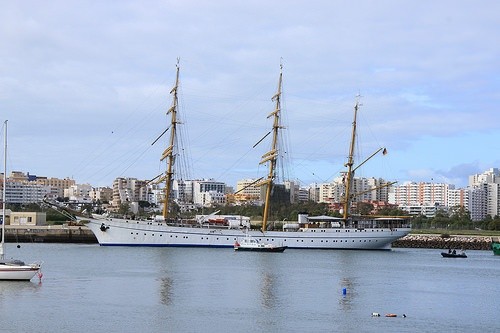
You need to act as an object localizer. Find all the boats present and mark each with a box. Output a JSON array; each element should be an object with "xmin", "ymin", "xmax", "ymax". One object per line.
[
  {"xmin": 235, "ymin": 235, "xmax": 288, "ymax": 253},
  {"xmin": 441, "ymin": 252, "xmax": 467, "ymax": 258}
]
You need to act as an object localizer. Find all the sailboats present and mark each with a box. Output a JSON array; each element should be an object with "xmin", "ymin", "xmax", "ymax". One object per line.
[
  {"xmin": 42, "ymin": 55, "xmax": 412, "ymax": 251},
  {"xmin": 0, "ymin": 119, "xmax": 44, "ymax": 281}
]
